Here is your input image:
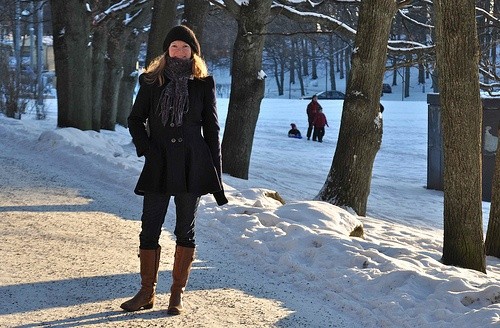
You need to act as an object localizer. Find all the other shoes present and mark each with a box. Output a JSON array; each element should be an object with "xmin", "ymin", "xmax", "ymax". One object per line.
[{"xmin": 307, "ymin": 137, "xmax": 310, "ymax": 140}]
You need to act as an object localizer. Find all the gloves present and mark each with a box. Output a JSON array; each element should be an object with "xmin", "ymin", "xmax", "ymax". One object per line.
[{"xmin": 137, "ymin": 136, "xmax": 162, "ymax": 162}]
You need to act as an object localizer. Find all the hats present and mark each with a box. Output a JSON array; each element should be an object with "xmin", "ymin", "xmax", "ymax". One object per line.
[
  {"xmin": 162, "ymin": 25, "xmax": 201, "ymax": 56},
  {"xmin": 291, "ymin": 123, "xmax": 295, "ymax": 126}
]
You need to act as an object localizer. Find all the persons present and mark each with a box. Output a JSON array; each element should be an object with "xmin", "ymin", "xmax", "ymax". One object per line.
[
  {"xmin": 287, "ymin": 123, "xmax": 302, "ymax": 139},
  {"xmin": 120, "ymin": 25, "xmax": 228, "ymax": 316},
  {"xmin": 307, "ymin": 95, "xmax": 329, "ymax": 142}
]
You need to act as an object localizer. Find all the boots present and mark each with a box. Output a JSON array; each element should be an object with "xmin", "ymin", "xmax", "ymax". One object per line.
[
  {"xmin": 120, "ymin": 246, "xmax": 161, "ymax": 312},
  {"xmin": 167, "ymin": 245, "xmax": 195, "ymax": 315}
]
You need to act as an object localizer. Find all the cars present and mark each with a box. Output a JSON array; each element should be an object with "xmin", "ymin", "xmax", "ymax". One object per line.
[
  {"xmin": 383, "ymin": 83, "xmax": 392, "ymax": 94},
  {"xmin": 300, "ymin": 90, "xmax": 345, "ymax": 100}
]
